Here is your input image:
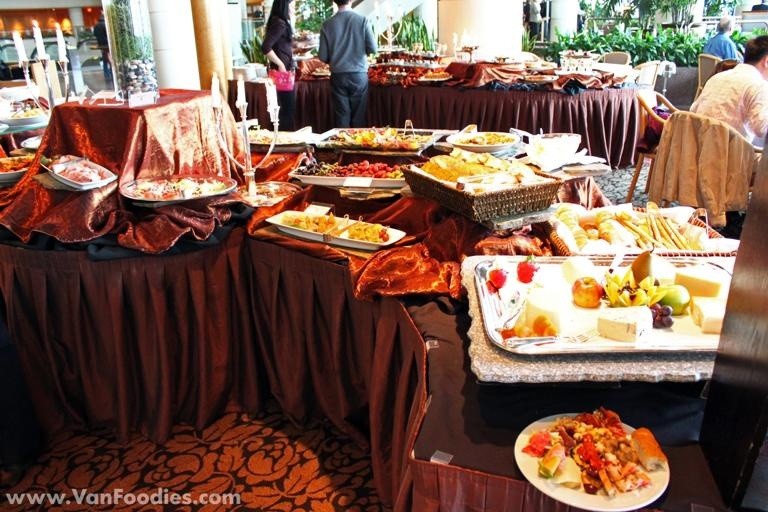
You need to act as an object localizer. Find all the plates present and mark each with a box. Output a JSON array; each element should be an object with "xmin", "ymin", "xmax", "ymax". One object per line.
[
  {"xmin": 20, "ymin": 136, "xmax": 44, "ymax": 148},
  {"xmin": 265, "ymin": 209, "xmax": 405, "ymax": 251},
  {"xmin": 0, "ymin": 115, "xmax": 48, "ymax": 127},
  {"xmin": 417, "ymin": 76, "xmax": 453, "ymax": 84},
  {"xmin": 312, "ymin": 72, "xmax": 330, "ymax": 76},
  {"xmin": 119, "ymin": 177, "xmax": 238, "ymax": 201},
  {"xmin": 518, "ymin": 75, "xmax": 559, "ymax": 83},
  {"xmin": 236, "ymin": 181, "xmax": 303, "ymax": 208},
  {"xmin": 0, "ymin": 156, "xmax": 32, "ymax": 182},
  {"xmin": 41, "ymin": 155, "xmax": 118, "ymax": 188},
  {"xmin": 445, "ymin": 132, "xmax": 521, "ymax": 153},
  {"xmin": 521, "ymin": 63, "xmax": 559, "ymax": 70},
  {"xmin": 514, "ymin": 413, "xmax": 671, "ymax": 511}
]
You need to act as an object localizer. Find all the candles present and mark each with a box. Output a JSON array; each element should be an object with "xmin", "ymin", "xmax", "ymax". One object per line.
[
  {"xmin": 13, "ymin": 30, "xmax": 26, "ymax": 60},
  {"xmin": 235, "ymin": 73, "xmax": 246, "ymax": 105},
  {"xmin": 212, "ymin": 72, "xmax": 220, "ymax": 106},
  {"xmin": 266, "ymin": 79, "xmax": 279, "ymax": 108},
  {"xmin": 54, "ymin": 23, "xmax": 67, "ymax": 60},
  {"xmin": 32, "ymin": 21, "xmax": 47, "ymax": 56}
]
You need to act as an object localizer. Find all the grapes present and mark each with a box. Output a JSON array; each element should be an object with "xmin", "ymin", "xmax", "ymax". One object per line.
[{"xmin": 652, "ymin": 304, "xmax": 673, "ymax": 330}]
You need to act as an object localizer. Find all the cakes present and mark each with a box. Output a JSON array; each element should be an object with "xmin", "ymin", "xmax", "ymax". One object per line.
[
  {"xmin": 515, "ymin": 299, "xmax": 560, "ymax": 339},
  {"xmin": 595, "ymin": 306, "xmax": 654, "ymax": 343}
]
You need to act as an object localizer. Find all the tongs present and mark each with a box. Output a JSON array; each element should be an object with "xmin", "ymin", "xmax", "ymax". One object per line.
[
  {"xmin": 52, "ymin": 156, "xmax": 86, "ymax": 172},
  {"xmin": 457, "ymin": 172, "xmax": 526, "ymax": 190},
  {"xmin": 645, "ymin": 201, "xmax": 683, "ymax": 250},
  {"xmin": 678, "ymin": 208, "xmax": 710, "ymax": 250},
  {"xmin": 402, "ymin": 119, "xmax": 416, "ymax": 139}
]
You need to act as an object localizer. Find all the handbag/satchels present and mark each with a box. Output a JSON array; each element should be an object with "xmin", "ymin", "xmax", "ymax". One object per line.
[
  {"xmin": 636, "ymin": 106, "xmax": 672, "ymax": 153},
  {"xmin": 268, "ymin": 66, "xmax": 296, "ymax": 92}
]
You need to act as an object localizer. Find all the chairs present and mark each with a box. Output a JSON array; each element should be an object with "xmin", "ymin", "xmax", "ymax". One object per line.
[
  {"xmin": 626, "ymin": 90, "xmax": 680, "ymax": 203},
  {"xmin": 653, "ymin": 111, "xmax": 764, "ymax": 239},
  {"xmin": 635, "ymin": 61, "xmax": 660, "ymax": 90},
  {"xmin": 595, "ymin": 52, "xmax": 632, "ymax": 66},
  {"xmin": 693, "ymin": 53, "xmax": 724, "ymax": 102}
]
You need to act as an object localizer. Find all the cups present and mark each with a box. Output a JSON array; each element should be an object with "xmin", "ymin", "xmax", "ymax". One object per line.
[
  {"xmin": 578, "ymin": 60, "xmax": 585, "ymax": 71},
  {"xmin": 569, "ymin": 59, "xmax": 577, "ymax": 72},
  {"xmin": 456, "ymin": 50, "xmax": 464, "ymax": 60},
  {"xmin": 411, "ymin": 43, "xmax": 423, "ymax": 55},
  {"xmin": 560, "ymin": 59, "xmax": 568, "ymax": 72},
  {"xmin": 586, "ymin": 60, "xmax": 593, "ymax": 73},
  {"xmin": 433, "ymin": 42, "xmax": 447, "ymax": 58}
]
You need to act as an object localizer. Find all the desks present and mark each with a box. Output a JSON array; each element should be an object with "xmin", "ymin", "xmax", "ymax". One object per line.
[
  {"xmin": 1, "ymin": 128, "xmax": 768, "ymax": 511},
  {"xmin": 34, "ymin": 88, "xmax": 251, "ymax": 189},
  {"xmin": 228, "ymin": 59, "xmax": 638, "ymax": 169}
]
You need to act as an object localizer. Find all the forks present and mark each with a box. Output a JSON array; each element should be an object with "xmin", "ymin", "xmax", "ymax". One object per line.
[{"xmin": 507, "ymin": 329, "xmax": 601, "ymax": 349}]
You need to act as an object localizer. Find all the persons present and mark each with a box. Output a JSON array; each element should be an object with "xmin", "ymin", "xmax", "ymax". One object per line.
[
  {"xmin": 702, "ymin": 18, "xmax": 737, "ymax": 61},
  {"xmin": 529, "ymin": 0, "xmax": 541, "ymax": 41},
  {"xmin": 262, "ymin": 0, "xmax": 296, "ymax": 131},
  {"xmin": 94, "ymin": 13, "xmax": 111, "ymax": 79},
  {"xmin": 689, "ymin": 35, "xmax": 768, "ymax": 143},
  {"xmin": 318, "ymin": 0, "xmax": 377, "ymax": 127}
]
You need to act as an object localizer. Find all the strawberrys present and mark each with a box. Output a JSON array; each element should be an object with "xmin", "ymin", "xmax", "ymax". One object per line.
[
  {"xmin": 519, "ymin": 261, "xmax": 536, "ymax": 282},
  {"xmin": 489, "ymin": 268, "xmax": 508, "ymax": 288}
]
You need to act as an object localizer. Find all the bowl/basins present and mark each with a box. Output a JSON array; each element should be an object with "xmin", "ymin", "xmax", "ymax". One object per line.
[{"xmin": 529, "ymin": 133, "xmax": 582, "ymax": 156}]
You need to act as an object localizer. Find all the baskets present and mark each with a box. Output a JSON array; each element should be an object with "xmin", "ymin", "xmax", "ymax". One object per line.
[{"xmin": 400, "ymin": 161, "xmax": 562, "ymax": 223}]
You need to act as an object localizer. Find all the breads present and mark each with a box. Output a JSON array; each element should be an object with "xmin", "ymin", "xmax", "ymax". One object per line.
[
  {"xmin": 633, "ymin": 427, "xmax": 669, "ymax": 470},
  {"xmin": 556, "ymin": 201, "xmax": 698, "ymax": 254},
  {"xmin": 693, "ymin": 297, "xmax": 725, "ymax": 333}
]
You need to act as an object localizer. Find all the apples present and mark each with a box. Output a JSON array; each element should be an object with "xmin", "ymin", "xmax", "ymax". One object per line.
[
  {"xmin": 663, "ymin": 286, "xmax": 690, "ymax": 314},
  {"xmin": 574, "ymin": 276, "xmax": 602, "ymax": 307}
]
